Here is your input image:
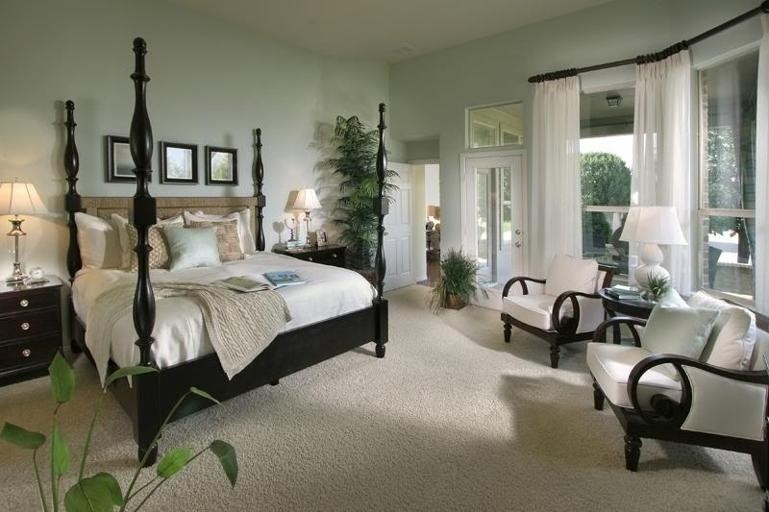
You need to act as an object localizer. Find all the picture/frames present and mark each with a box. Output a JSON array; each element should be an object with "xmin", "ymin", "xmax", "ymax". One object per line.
[
  {"xmin": 107, "ymin": 135, "xmax": 153, "ymax": 183},
  {"xmin": 205, "ymin": 145, "xmax": 238, "ymax": 186},
  {"xmin": 160, "ymin": 140, "xmax": 198, "ymax": 185}
]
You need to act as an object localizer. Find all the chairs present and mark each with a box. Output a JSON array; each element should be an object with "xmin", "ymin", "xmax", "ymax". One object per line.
[{"xmin": 501, "ymin": 262, "xmax": 619, "ymax": 368}]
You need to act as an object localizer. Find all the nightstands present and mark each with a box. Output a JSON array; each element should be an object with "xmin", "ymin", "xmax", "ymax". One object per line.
[
  {"xmin": 0, "ymin": 275, "xmax": 64, "ymax": 388},
  {"xmin": 271, "ymin": 241, "xmax": 347, "ymax": 267}
]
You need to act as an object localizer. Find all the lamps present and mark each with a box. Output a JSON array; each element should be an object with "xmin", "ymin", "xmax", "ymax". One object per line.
[
  {"xmin": 0, "ymin": 182, "xmax": 48, "ymax": 282},
  {"xmin": 286, "ymin": 188, "xmax": 323, "ymax": 249},
  {"xmin": 605, "ymin": 94, "xmax": 624, "ymax": 108},
  {"xmin": 618, "ymin": 206, "xmax": 688, "ymax": 305}
]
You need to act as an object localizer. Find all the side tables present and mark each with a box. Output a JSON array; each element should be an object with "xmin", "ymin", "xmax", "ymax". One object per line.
[{"xmin": 599, "ymin": 286, "xmax": 697, "ymax": 344}]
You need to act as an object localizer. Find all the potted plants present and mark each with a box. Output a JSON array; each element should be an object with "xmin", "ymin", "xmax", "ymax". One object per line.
[
  {"xmin": 640, "ymin": 269, "xmax": 670, "ymax": 301},
  {"xmin": 327, "ymin": 115, "xmax": 401, "ymax": 286},
  {"xmin": 424, "ymin": 247, "xmax": 489, "ymax": 316}
]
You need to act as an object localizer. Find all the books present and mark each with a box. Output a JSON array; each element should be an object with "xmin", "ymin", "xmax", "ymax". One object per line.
[
  {"xmin": 613, "ymin": 284, "xmax": 647, "ymax": 295},
  {"xmin": 214, "ymin": 275, "xmax": 271, "ymax": 293},
  {"xmin": 604, "ymin": 288, "xmax": 640, "ymax": 300},
  {"xmin": 262, "ymin": 270, "xmax": 307, "ymax": 286}
]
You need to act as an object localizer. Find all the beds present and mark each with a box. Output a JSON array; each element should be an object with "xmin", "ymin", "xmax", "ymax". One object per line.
[{"xmin": 64, "ymin": 36, "xmax": 389, "ymax": 467}]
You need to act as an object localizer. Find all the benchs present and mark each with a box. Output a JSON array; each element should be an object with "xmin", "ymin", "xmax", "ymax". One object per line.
[{"xmin": 585, "ymin": 298, "xmax": 769, "ymax": 492}]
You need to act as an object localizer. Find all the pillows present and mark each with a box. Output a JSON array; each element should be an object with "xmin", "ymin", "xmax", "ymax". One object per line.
[
  {"xmin": 74, "ymin": 209, "xmax": 256, "ymax": 272},
  {"xmin": 642, "ymin": 288, "xmax": 720, "ymax": 382},
  {"xmin": 545, "ymin": 254, "xmax": 598, "ymax": 300},
  {"xmin": 687, "ymin": 288, "xmax": 757, "ymax": 373}
]
